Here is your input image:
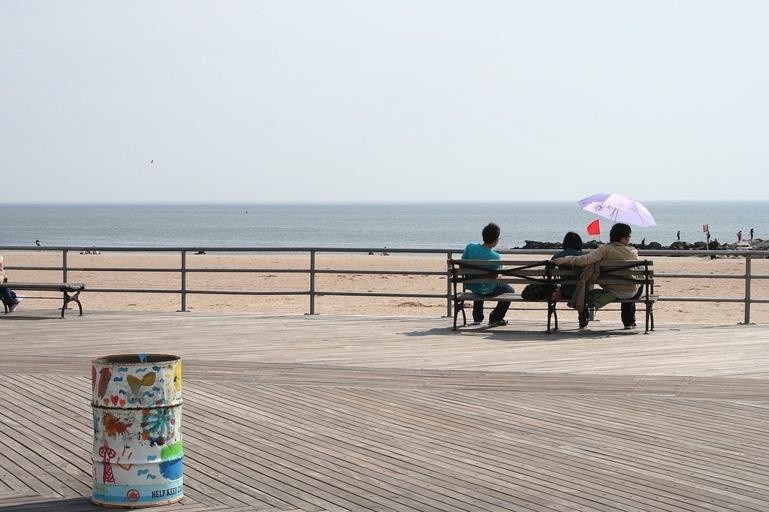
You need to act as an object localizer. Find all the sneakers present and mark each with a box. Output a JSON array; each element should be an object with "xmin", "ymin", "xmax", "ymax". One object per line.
[
  {"xmin": 580, "ymin": 325, "xmax": 591, "ymax": 330},
  {"xmin": 474, "ymin": 319, "xmax": 481, "ymax": 325},
  {"xmin": 624, "ymin": 323, "xmax": 637, "ymax": 329},
  {"xmin": 489, "ymin": 318, "xmax": 508, "ymax": 327}
]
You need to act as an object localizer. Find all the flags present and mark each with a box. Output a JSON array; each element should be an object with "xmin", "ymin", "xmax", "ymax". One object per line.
[{"xmin": 587, "ymin": 220, "xmax": 600, "ymax": 235}]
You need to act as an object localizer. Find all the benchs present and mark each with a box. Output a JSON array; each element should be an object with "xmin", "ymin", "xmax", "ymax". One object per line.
[
  {"xmin": 0, "ymin": 282, "xmax": 85, "ymax": 320},
  {"xmin": 448, "ymin": 260, "xmax": 659, "ymax": 335}
]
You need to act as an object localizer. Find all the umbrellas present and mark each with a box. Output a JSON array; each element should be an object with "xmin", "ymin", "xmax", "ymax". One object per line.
[{"xmin": 578, "ymin": 192, "xmax": 657, "ymax": 228}]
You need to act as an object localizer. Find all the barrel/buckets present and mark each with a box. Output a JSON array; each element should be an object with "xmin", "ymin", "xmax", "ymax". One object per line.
[{"xmin": 90, "ymin": 355, "xmax": 183, "ymax": 507}]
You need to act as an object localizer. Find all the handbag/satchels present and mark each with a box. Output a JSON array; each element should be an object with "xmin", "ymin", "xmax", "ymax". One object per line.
[
  {"xmin": 521, "ymin": 283, "xmax": 556, "ymax": 299},
  {"xmin": 586, "ymin": 289, "xmax": 616, "ymax": 308}
]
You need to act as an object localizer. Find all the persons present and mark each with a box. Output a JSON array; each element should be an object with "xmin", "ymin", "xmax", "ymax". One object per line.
[
  {"xmin": 549, "ymin": 233, "xmax": 589, "ymax": 329},
  {"xmin": 0, "ymin": 256, "xmax": 24, "ymax": 312},
  {"xmin": 551, "ymin": 224, "xmax": 644, "ymax": 329},
  {"xmin": 677, "ymin": 228, "xmax": 754, "ymax": 260},
  {"xmin": 461, "ymin": 223, "xmax": 514, "ymax": 326}
]
